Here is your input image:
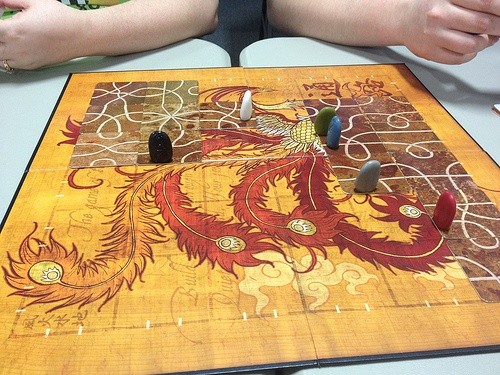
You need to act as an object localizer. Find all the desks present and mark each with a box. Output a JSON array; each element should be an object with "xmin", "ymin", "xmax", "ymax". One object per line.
[
  {"xmin": 0, "ymin": 36, "xmax": 276, "ymax": 375},
  {"xmin": 240, "ymin": 38, "xmax": 500, "ymax": 375}
]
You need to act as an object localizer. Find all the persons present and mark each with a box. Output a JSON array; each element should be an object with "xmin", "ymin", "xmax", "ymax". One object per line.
[
  {"xmin": 265, "ymin": 0, "xmax": 500, "ymax": 66},
  {"xmin": 0, "ymin": 0, "xmax": 220, "ymax": 75}
]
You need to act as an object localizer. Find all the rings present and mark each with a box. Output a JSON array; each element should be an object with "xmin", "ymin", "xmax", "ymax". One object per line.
[{"xmin": 3, "ymin": 60, "xmax": 14, "ymax": 71}]
